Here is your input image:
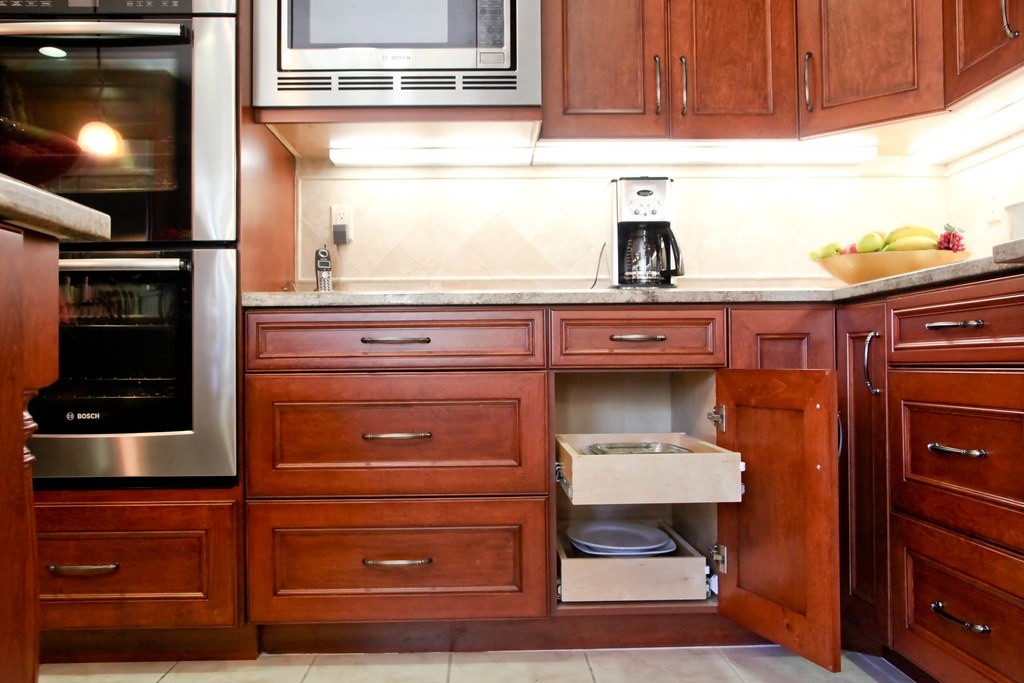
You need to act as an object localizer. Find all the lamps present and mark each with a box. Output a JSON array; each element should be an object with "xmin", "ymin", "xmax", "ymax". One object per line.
[{"xmin": 76, "ymin": 48, "xmax": 125, "ymax": 157}]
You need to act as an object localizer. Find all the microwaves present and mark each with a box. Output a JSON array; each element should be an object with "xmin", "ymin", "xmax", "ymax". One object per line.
[{"xmin": 248, "ymin": 0, "xmax": 543, "ymax": 108}]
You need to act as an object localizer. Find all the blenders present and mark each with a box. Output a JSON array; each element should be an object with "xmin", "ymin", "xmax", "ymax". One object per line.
[{"xmin": 608, "ymin": 176, "xmax": 685, "ymax": 290}]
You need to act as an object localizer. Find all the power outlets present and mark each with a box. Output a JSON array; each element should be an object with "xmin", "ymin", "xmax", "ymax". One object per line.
[{"xmin": 331, "ymin": 203, "xmax": 354, "ymax": 241}]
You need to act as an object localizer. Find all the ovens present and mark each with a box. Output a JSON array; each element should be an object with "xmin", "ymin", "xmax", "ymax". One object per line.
[{"xmin": 0, "ymin": 0, "xmax": 239, "ymax": 491}]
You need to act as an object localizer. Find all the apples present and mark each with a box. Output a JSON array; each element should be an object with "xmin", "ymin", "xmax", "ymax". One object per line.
[{"xmin": 820, "ymin": 233, "xmax": 883, "ymax": 259}]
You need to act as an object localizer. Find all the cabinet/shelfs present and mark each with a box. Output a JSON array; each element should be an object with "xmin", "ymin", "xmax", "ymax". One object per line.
[
  {"xmin": 834, "ymin": 270, "xmax": 1024, "ymax": 683},
  {"xmin": 32, "ymin": 489, "xmax": 262, "ymax": 666},
  {"xmin": 531, "ymin": 0, "xmax": 799, "ymax": 167},
  {"xmin": 797, "ymin": 0, "xmax": 949, "ymax": 167},
  {"xmin": 945, "ymin": 0, "xmax": 1024, "ymax": 164},
  {"xmin": 241, "ymin": 308, "xmax": 843, "ymax": 672},
  {"xmin": 727, "ymin": 303, "xmax": 850, "ymax": 647}
]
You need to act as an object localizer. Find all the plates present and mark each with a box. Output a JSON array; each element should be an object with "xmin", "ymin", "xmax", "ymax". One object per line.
[
  {"xmin": 580, "ymin": 442, "xmax": 693, "ymax": 455},
  {"xmin": 817, "ymin": 249, "xmax": 970, "ymax": 285},
  {"xmin": 566, "ymin": 520, "xmax": 676, "ymax": 558}
]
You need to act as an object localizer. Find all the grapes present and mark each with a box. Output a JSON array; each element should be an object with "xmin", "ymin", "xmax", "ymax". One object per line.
[{"xmin": 938, "ymin": 231, "xmax": 965, "ymax": 253}]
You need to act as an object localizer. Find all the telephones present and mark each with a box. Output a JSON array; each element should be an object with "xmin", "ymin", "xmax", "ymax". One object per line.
[{"xmin": 315, "ymin": 245, "xmax": 333, "ymax": 291}]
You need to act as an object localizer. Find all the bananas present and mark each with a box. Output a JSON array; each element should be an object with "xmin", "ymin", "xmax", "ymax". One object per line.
[{"xmin": 882, "ymin": 226, "xmax": 939, "ymax": 251}]
